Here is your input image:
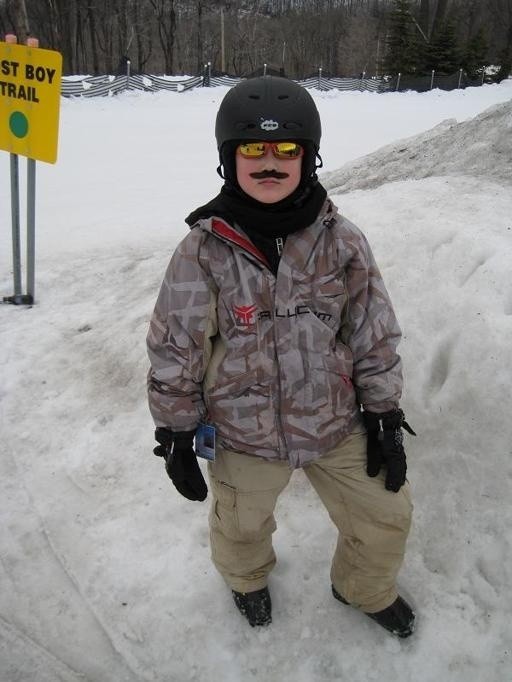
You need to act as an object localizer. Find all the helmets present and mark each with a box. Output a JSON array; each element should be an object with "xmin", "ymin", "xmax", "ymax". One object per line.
[{"xmin": 214, "ymin": 74, "xmax": 321, "ymax": 155}]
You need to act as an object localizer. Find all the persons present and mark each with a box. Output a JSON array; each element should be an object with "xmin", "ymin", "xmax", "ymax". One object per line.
[{"xmin": 140, "ymin": 62, "xmax": 419, "ymax": 644}]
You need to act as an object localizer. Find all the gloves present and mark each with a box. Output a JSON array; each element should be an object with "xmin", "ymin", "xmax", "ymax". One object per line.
[
  {"xmin": 152, "ymin": 426, "xmax": 208, "ymax": 502},
  {"xmin": 362, "ymin": 408, "xmax": 418, "ymax": 494}
]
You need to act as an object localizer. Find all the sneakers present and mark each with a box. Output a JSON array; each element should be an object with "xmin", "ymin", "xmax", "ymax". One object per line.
[
  {"xmin": 331, "ymin": 583, "xmax": 416, "ymax": 639},
  {"xmin": 231, "ymin": 584, "xmax": 273, "ymax": 627}
]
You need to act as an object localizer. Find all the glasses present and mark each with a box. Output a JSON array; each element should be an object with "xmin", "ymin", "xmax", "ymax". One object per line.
[{"xmin": 238, "ymin": 142, "xmax": 305, "ymax": 161}]
